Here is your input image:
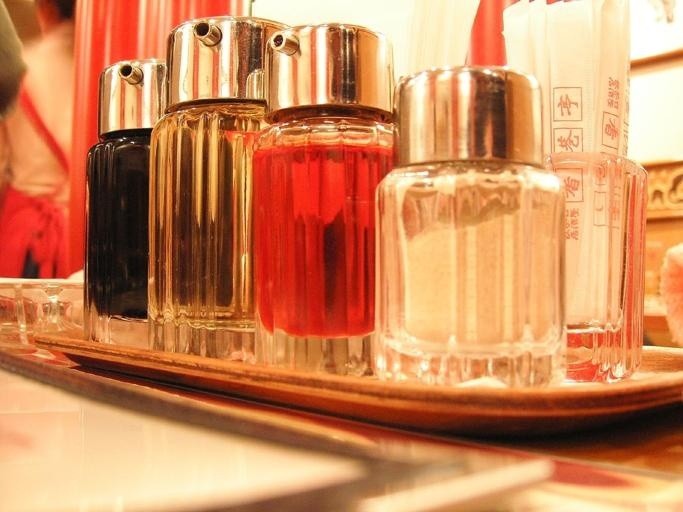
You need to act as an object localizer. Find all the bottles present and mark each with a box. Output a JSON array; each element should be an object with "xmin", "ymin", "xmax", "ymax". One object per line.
[
  {"xmin": 375, "ymin": 64, "xmax": 565, "ymax": 399},
  {"xmin": 150, "ymin": 14, "xmax": 289, "ymax": 369},
  {"xmin": 86, "ymin": 58, "xmax": 189, "ymax": 359},
  {"xmin": 251, "ymin": 23, "xmax": 392, "ymax": 380}
]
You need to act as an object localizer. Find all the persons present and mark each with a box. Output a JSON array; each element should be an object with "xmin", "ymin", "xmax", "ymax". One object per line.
[{"xmin": 0, "ymin": 3, "xmax": 76, "ymax": 282}]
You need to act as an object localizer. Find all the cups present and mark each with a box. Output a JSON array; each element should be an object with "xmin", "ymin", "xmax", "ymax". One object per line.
[{"xmin": 536, "ymin": 153, "xmax": 644, "ymax": 388}]
